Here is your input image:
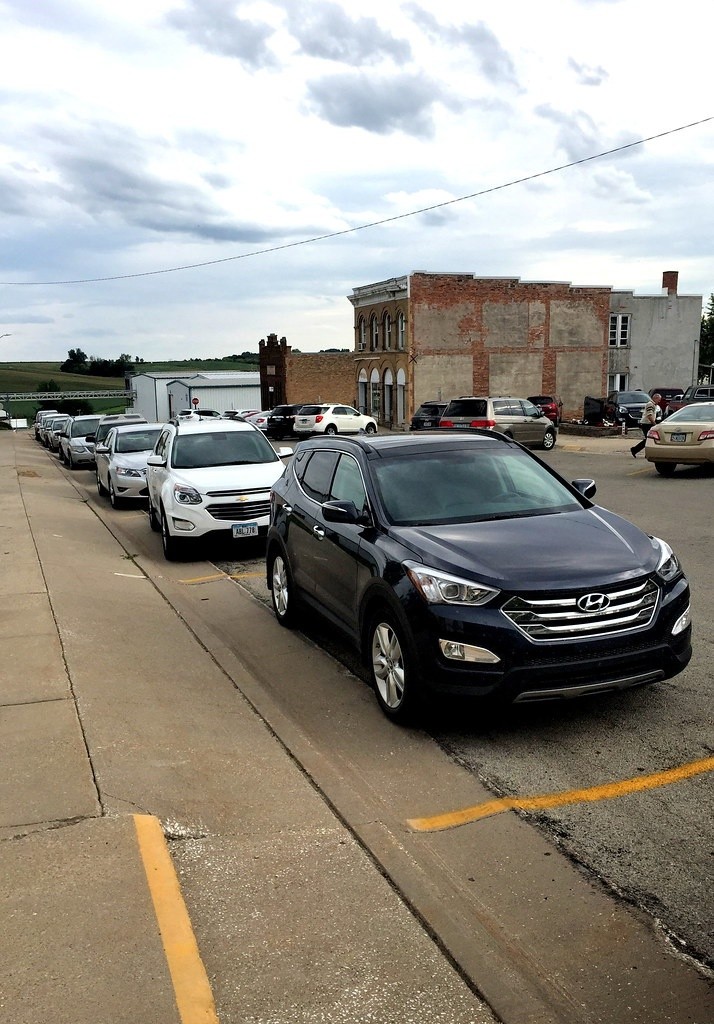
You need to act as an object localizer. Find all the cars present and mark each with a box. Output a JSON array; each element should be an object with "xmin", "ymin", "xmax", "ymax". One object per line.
[
  {"xmin": 648, "ymin": 385, "xmax": 684, "ymax": 417},
  {"xmin": 669, "ymin": 385, "xmax": 714, "ymax": 416},
  {"xmin": 96, "ymin": 422, "xmax": 169, "ymax": 508},
  {"xmin": 584, "ymin": 388, "xmax": 662, "ymax": 428},
  {"xmin": 644, "ymin": 402, "xmax": 714, "ymax": 475},
  {"xmin": 527, "ymin": 394, "xmax": 563, "ymax": 425},
  {"xmin": 36, "ymin": 404, "xmax": 148, "ymax": 468}
]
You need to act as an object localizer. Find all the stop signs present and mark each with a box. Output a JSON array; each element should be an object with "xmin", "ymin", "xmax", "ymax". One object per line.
[{"xmin": 192, "ymin": 397, "xmax": 199, "ymax": 404}]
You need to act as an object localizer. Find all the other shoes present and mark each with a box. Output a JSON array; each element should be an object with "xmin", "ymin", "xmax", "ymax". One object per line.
[{"xmin": 629, "ymin": 446, "xmax": 636, "ymax": 458}]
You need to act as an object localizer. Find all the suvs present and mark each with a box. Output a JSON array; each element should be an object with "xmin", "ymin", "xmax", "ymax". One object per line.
[
  {"xmin": 409, "ymin": 401, "xmax": 450, "ymax": 430},
  {"xmin": 293, "ymin": 403, "xmax": 378, "ymax": 435},
  {"xmin": 269, "ymin": 403, "xmax": 324, "ymax": 440},
  {"xmin": 263, "ymin": 426, "xmax": 697, "ymax": 729},
  {"xmin": 439, "ymin": 394, "xmax": 557, "ymax": 451},
  {"xmin": 148, "ymin": 416, "xmax": 295, "ymax": 563}
]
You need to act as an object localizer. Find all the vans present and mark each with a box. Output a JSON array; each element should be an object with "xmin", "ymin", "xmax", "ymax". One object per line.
[{"xmin": 177, "ymin": 409, "xmax": 273, "ymax": 437}]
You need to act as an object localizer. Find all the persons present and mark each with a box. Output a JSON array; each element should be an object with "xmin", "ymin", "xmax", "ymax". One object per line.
[{"xmin": 631, "ymin": 393, "xmax": 661, "ymax": 458}]
